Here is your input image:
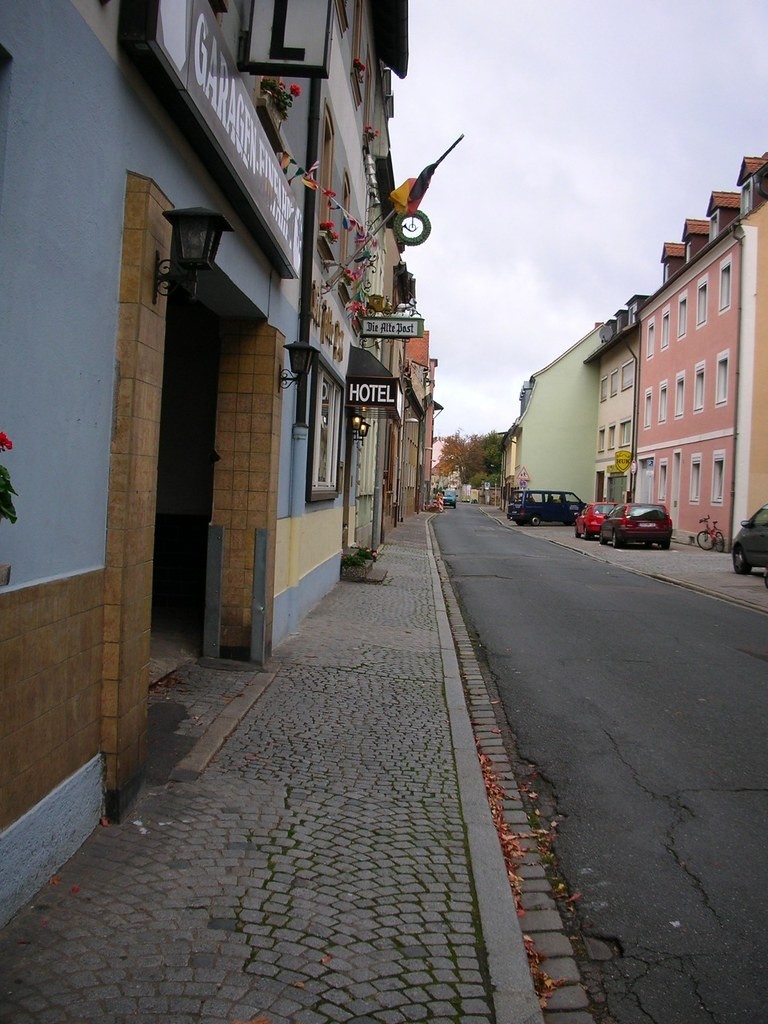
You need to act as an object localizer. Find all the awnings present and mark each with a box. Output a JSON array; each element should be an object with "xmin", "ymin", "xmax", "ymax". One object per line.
[
  {"xmin": 369, "ymin": 0, "xmax": 408, "ymax": 79},
  {"xmin": 433, "ymin": 401, "xmax": 444, "ymax": 419}
]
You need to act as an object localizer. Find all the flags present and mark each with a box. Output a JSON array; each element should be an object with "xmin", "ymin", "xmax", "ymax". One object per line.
[
  {"xmin": 281, "ymin": 150, "xmax": 365, "ymax": 239},
  {"xmin": 388, "ymin": 164, "xmax": 436, "ymax": 215}
]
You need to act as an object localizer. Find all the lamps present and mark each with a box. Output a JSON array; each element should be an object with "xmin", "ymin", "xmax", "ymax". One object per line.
[
  {"xmin": 347, "ymin": 414, "xmax": 371, "ymax": 447},
  {"xmin": 152, "ymin": 205, "xmax": 235, "ymax": 306},
  {"xmin": 278, "ymin": 341, "xmax": 322, "ymax": 394},
  {"xmin": 363, "ymin": 145, "xmax": 381, "ymax": 207}
]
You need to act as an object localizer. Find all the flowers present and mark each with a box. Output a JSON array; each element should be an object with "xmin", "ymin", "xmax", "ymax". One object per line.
[
  {"xmin": 318, "ymin": 222, "xmax": 339, "ymax": 245},
  {"xmin": 341, "ymin": 269, "xmax": 356, "ymax": 288},
  {"xmin": 363, "ymin": 125, "xmax": 379, "ymax": 142},
  {"xmin": 260, "ymin": 78, "xmax": 303, "ymax": 121},
  {"xmin": 352, "ymin": 57, "xmax": 366, "ymax": 85},
  {"xmin": 0, "ymin": 431, "xmax": 22, "ymax": 526}
]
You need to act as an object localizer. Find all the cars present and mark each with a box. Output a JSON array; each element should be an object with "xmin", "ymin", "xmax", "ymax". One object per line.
[
  {"xmin": 732, "ymin": 504, "xmax": 768, "ymax": 576},
  {"xmin": 599, "ymin": 503, "xmax": 673, "ymax": 549},
  {"xmin": 573, "ymin": 501, "xmax": 620, "ymax": 540}
]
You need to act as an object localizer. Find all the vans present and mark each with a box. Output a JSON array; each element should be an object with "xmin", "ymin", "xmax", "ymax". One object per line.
[
  {"xmin": 507, "ymin": 489, "xmax": 587, "ymax": 527},
  {"xmin": 442, "ymin": 489, "xmax": 458, "ymax": 509}
]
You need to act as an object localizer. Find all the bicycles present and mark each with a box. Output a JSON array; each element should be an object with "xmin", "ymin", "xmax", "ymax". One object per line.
[{"xmin": 697, "ymin": 514, "xmax": 725, "ymax": 552}]
